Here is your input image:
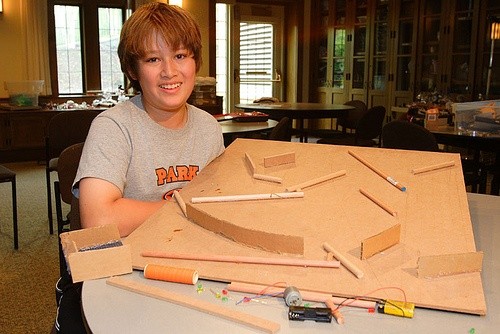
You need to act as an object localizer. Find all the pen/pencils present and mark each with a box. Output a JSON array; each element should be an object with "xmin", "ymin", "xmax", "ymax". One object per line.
[{"xmin": 348, "ymin": 149, "xmax": 406, "ymax": 192}]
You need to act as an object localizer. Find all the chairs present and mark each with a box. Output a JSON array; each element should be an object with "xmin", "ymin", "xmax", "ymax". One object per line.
[
  {"xmin": 267, "ymin": 117, "xmax": 294, "ymax": 141},
  {"xmin": 45, "ymin": 111, "xmax": 102, "ymax": 235},
  {"xmin": 313, "ymin": 99, "xmax": 386, "ymax": 148},
  {"xmin": 0, "ymin": 147, "xmax": 18, "ymax": 249},
  {"xmin": 58, "ymin": 141, "xmax": 84, "ymax": 278}
]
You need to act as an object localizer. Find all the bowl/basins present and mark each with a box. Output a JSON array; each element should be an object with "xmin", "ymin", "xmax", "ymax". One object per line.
[{"xmin": 4, "ymin": 81, "xmax": 44, "ymax": 107}]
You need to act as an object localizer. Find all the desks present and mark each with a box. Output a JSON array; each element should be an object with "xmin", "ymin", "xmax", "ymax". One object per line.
[
  {"xmin": 214, "ymin": 114, "xmax": 278, "ymax": 147},
  {"xmin": 82, "ymin": 191, "xmax": 500, "ymax": 334},
  {"xmin": 235, "ymin": 102, "xmax": 356, "ymax": 143},
  {"xmin": 0, "ymin": 98, "xmax": 223, "ymax": 160}
]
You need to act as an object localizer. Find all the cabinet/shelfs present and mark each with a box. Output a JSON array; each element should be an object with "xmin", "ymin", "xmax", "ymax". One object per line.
[{"xmin": 310, "ymin": 0, "xmax": 500, "ymax": 137}]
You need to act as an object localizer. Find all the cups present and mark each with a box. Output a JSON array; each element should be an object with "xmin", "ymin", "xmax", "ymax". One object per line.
[{"xmin": 455, "ymin": 110, "xmax": 477, "ymax": 136}]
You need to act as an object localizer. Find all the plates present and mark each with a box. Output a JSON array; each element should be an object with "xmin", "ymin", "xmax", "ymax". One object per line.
[{"xmin": 232, "ymin": 112, "xmax": 269, "ymax": 123}]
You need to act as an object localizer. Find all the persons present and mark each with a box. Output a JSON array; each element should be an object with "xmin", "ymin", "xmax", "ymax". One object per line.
[{"xmin": 71, "ymin": 1, "xmax": 226, "ymax": 239}]
